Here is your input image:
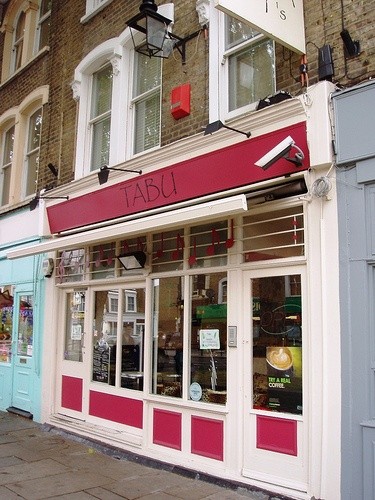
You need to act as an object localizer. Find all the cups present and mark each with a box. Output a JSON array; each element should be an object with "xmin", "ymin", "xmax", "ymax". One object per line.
[{"xmin": 266, "ymin": 347, "xmax": 294, "ymax": 380}]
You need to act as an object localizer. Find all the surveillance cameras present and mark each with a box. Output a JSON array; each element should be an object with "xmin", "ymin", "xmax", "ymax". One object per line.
[{"xmin": 253, "ymin": 135, "xmax": 295, "ymax": 171}]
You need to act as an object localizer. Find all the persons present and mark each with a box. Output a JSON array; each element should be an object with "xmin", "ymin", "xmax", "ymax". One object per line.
[{"xmin": 117, "ymin": 324, "xmax": 134, "ymax": 347}]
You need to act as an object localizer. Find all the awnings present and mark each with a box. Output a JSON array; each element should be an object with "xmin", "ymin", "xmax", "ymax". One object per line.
[{"xmin": 6, "ymin": 180, "xmax": 304, "ymax": 262}]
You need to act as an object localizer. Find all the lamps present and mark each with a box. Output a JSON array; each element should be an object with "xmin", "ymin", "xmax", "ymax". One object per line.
[
  {"xmin": 205, "ymin": 120, "xmax": 251, "ymax": 141},
  {"xmin": 127, "ymin": 1, "xmax": 203, "ymax": 67},
  {"xmin": 97, "ymin": 166, "xmax": 141, "ymax": 185},
  {"xmin": 29, "ymin": 196, "xmax": 67, "ymax": 210},
  {"xmin": 117, "ymin": 252, "xmax": 144, "ymax": 271}
]
